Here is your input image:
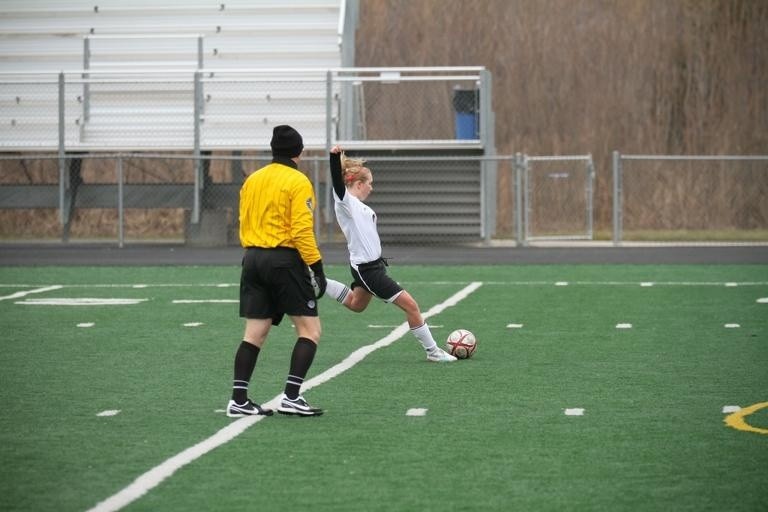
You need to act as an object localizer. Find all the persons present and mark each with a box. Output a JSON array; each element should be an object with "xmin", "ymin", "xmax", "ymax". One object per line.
[
  {"xmin": 226, "ymin": 125, "xmax": 328, "ymax": 417},
  {"xmin": 311, "ymin": 144, "xmax": 458, "ymax": 363}
]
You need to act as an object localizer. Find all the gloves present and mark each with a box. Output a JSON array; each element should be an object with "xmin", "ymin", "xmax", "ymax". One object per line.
[{"xmin": 313, "ymin": 272, "xmax": 326, "ymax": 299}]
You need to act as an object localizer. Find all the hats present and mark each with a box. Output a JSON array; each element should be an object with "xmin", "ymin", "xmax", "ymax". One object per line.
[{"xmin": 270, "ymin": 125, "xmax": 303, "ymax": 158}]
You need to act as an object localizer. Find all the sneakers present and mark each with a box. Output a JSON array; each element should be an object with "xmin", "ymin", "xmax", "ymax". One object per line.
[
  {"xmin": 226, "ymin": 399, "xmax": 273, "ymax": 418},
  {"xmin": 426, "ymin": 348, "xmax": 457, "ymax": 362},
  {"xmin": 276, "ymin": 391, "xmax": 323, "ymax": 416}
]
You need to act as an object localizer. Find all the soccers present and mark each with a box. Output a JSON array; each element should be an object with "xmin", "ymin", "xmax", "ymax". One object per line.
[{"xmin": 446, "ymin": 329, "xmax": 476, "ymax": 359}]
